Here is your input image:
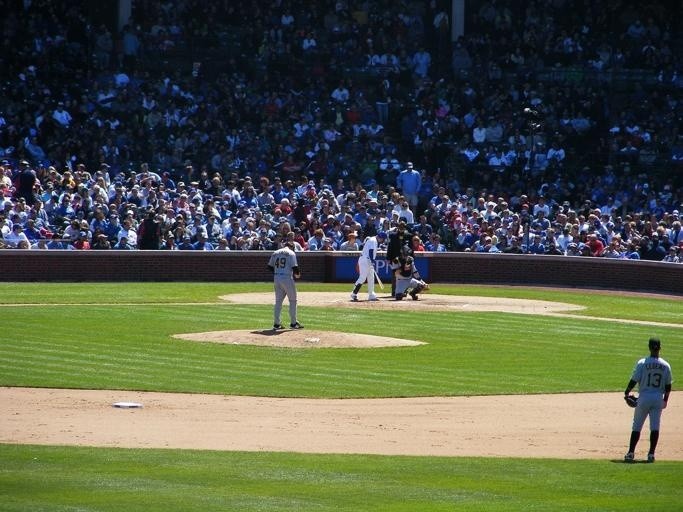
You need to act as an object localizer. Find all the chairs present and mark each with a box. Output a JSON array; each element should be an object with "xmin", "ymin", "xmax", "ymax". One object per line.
[
  {"xmin": 367, "ymin": 294, "xmax": 376, "ymax": 300},
  {"xmin": 624, "ymin": 452, "xmax": 633, "ymax": 462},
  {"xmin": 350, "ymin": 291, "xmax": 357, "ymax": 300},
  {"xmin": 395, "ymin": 292, "xmax": 417, "ymax": 300},
  {"xmin": 290, "ymin": 321, "xmax": 303, "ymax": 328},
  {"xmin": 647, "ymin": 454, "xmax": 654, "ymax": 463},
  {"xmin": 273, "ymin": 324, "xmax": 285, "ymax": 330}
]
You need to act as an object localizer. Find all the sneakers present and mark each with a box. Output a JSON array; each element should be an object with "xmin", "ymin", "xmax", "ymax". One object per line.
[
  {"xmin": 395, "ymin": 292, "xmax": 417, "ymax": 300},
  {"xmin": 647, "ymin": 454, "xmax": 654, "ymax": 463},
  {"xmin": 273, "ymin": 324, "xmax": 285, "ymax": 330},
  {"xmin": 290, "ymin": 321, "xmax": 303, "ymax": 328},
  {"xmin": 350, "ymin": 291, "xmax": 357, "ymax": 300},
  {"xmin": 367, "ymin": 294, "xmax": 376, "ymax": 300},
  {"xmin": 624, "ymin": 452, "xmax": 633, "ymax": 462}
]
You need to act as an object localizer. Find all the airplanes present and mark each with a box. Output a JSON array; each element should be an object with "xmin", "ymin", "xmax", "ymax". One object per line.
[{"xmin": 624, "ymin": 395, "xmax": 638, "ymax": 407}]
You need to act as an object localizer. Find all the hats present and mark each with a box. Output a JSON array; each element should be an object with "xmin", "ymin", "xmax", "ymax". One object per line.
[{"xmin": 2, "ymin": 83, "xmax": 682, "ymax": 258}]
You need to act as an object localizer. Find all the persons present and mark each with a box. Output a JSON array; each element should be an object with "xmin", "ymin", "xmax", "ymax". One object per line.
[
  {"xmin": 624, "ymin": 336, "xmax": 673, "ymax": 462},
  {"xmin": 386, "ymin": 221, "xmax": 429, "ymax": 301},
  {"xmin": 0, "ymin": 0, "xmax": 683, "ymax": 266},
  {"xmin": 266, "ymin": 243, "xmax": 303, "ymax": 330},
  {"xmin": 350, "ymin": 230, "xmax": 387, "ymax": 302}
]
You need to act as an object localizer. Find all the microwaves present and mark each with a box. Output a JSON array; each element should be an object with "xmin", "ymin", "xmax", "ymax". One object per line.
[{"xmin": 374, "ymin": 269, "xmax": 385, "ymax": 290}]
